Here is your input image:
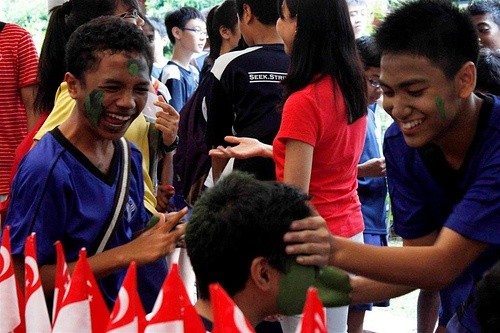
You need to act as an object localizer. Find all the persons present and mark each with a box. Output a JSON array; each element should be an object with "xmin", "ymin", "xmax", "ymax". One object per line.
[
  {"xmin": 1, "ymin": 1, "xmax": 500, "ymax": 310},
  {"xmin": 185, "ymin": 171, "xmax": 353, "ymax": 333},
  {"xmin": 6, "ymin": 17, "xmax": 189, "ymax": 315},
  {"xmin": 284, "ymin": 0, "xmax": 500, "ymax": 333},
  {"xmin": 209, "ymin": 0, "xmax": 367, "ymax": 333}
]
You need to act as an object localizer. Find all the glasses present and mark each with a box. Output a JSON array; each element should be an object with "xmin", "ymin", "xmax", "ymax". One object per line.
[
  {"xmin": 120, "ymin": 7, "xmax": 136, "ymax": 17},
  {"xmin": 180, "ymin": 25, "xmax": 207, "ymax": 34},
  {"xmin": 367, "ymin": 76, "xmax": 380, "ymax": 87}
]
